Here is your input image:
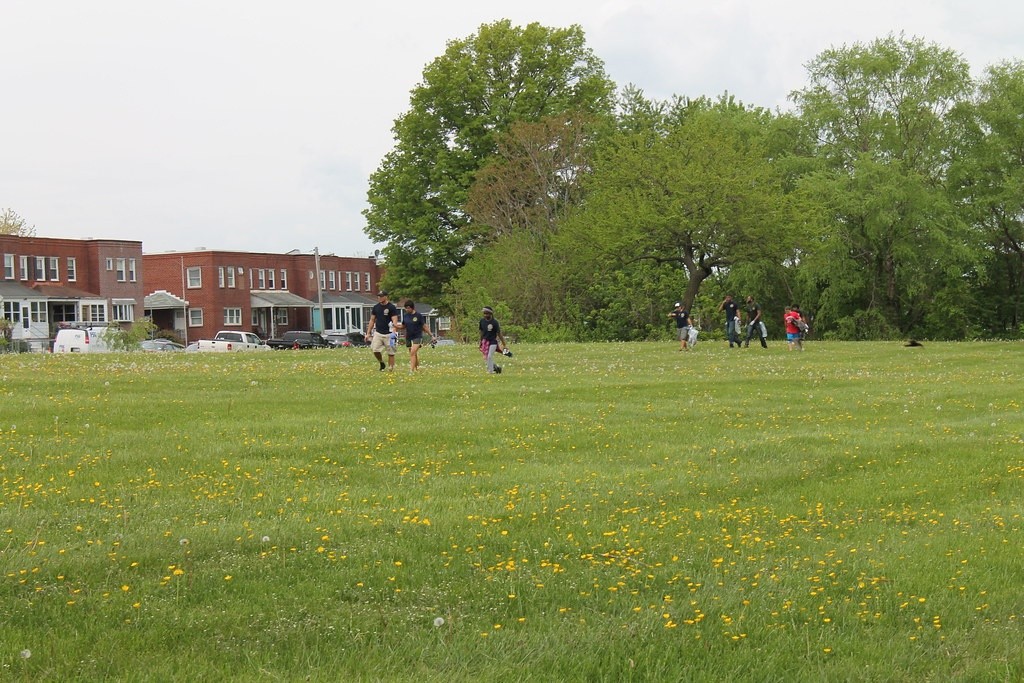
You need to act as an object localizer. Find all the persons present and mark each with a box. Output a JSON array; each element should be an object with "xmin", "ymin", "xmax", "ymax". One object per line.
[
  {"xmin": 364, "ymin": 291, "xmax": 398, "ymax": 370},
  {"xmin": 744, "ymin": 295, "xmax": 767, "ymax": 348},
  {"xmin": 793, "ymin": 305, "xmax": 806, "ymax": 350},
  {"xmin": 479, "ymin": 307, "xmax": 509, "ymax": 373},
  {"xmin": 719, "ymin": 295, "xmax": 742, "ymax": 348},
  {"xmin": 784, "ymin": 306, "xmax": 802, "ymax": 351},
  {"xmin": 667, "ymin": 303, "xmax": 692, "ymax": 350},
  {"xmin": 391, "ymin": 301, "xmax": 437, "ymax": 375}
]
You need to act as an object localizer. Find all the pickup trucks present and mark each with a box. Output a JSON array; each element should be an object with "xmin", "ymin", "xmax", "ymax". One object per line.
[
  {"xmin": 197, "ymin": 331, "xmax": 271, "ymax": 353},
  {"xmin": 266, "ymin": 331, "xmax": 328, "ymax": 350}
]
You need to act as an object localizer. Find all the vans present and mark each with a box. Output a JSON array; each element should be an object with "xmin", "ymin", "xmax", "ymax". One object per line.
[{"xmin": 54, "ymin": 323, "xmax": 145, "ymax": 354}]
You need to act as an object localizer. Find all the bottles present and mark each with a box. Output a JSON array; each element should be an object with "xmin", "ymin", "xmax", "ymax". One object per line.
[{"xmin": 389, "ymin": 321, "xmax": 393, "ymax": 332}]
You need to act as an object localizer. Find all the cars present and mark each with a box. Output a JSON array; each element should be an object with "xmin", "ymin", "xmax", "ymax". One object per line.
[
  {"xmin": 327, "ymin": 332, "xmax": 371, "ymax": 349},
  {"xmin": 142, "ymin": 339, "xmax": 187, "ymax": 354}
]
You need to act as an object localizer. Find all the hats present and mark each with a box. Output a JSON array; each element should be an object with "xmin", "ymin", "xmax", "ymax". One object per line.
[
  {"xmin": 674, "ymin": 302, "xmax": 681, "ymax": 309},
  {"xmin": 377, "ymin": 290, "xmax": 389, "ymax": 297},
  {"xmin": 481, "ymin": 308, "xmax": 493, "ymax": 313}
]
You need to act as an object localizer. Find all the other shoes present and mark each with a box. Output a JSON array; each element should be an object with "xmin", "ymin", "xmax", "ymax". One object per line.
[
  {"xmin": 762, "ymin": 344, "xmax": 767, "ymax": 348},
  {"xmin": 679, "ymin": 348, "xmax": 688, "ymax": 351},
  {"xmin": 379, "ymin": 362, "xmax": 386, "ymax": 371},
  {"xmin": 493, "ymin": 364, "xmax": 502, "ymax": 374},
  {"xmin": 388, "ymin": 365, "xmax": 394, "ymax": 372},
  {"xmin": 738, "ymin": 341, "xmax": 742, "ymax": 348},
  {"xmin": 744, "ymin": 344, "xmax": 749, "ymax": 347},
  {"xmin": 408, "ymin": 369, "xmax": 417, "ymax": 376},
  {"xmin": 730, "ymin": 343, "xmax": 734, "ymax": 347}
]
